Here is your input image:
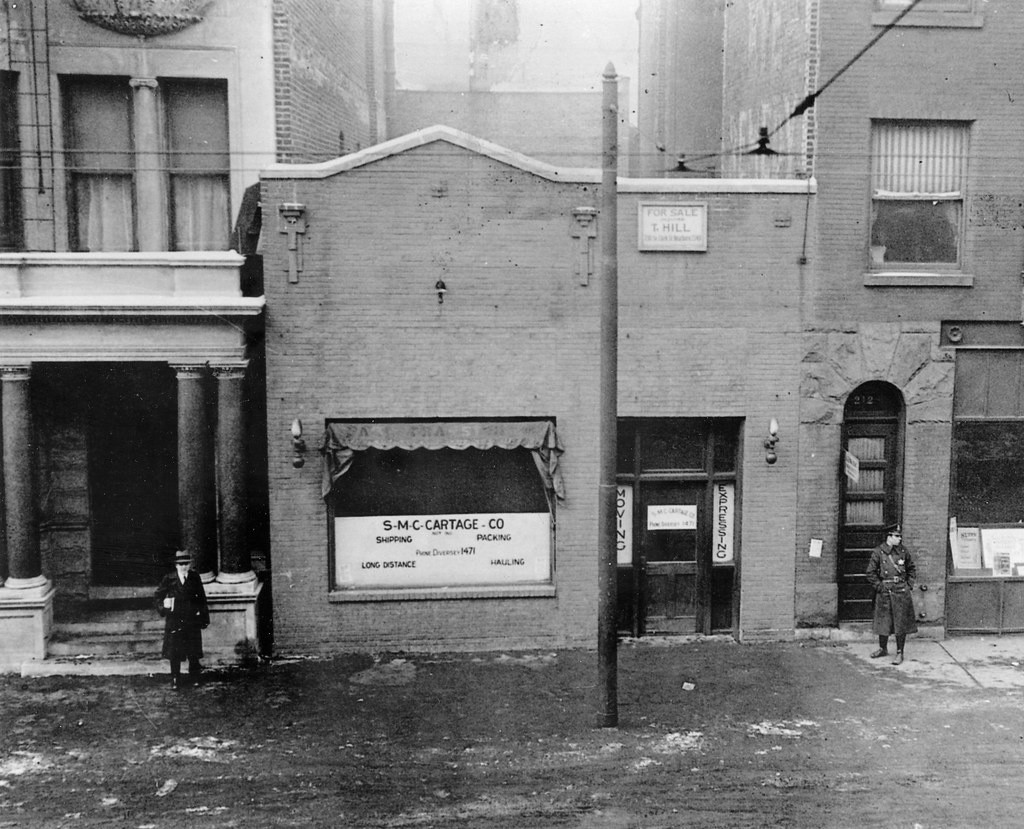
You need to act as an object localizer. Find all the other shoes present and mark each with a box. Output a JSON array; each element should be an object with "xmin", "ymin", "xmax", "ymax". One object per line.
[
  {"xmin": 190, "ymin": 675, "xmax": 199, "ymax": 686},
  {"xmin": 172, "ymin": 676, "xmax": 180, "ymax": 690}
]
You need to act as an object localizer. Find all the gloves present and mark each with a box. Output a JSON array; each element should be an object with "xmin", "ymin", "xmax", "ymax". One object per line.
[{"xmin": 164, "ymin": 607, "xmax": 172, "ymax": 617}]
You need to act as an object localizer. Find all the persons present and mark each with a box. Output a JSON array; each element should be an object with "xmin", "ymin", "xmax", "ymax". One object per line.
[
  {"xmin": 867, "ymin": 524, "xmax": 915, "ymax": 665},
  {"xmin": 152, "ymin": 549, "xmax": 210, "ymax": 691}
]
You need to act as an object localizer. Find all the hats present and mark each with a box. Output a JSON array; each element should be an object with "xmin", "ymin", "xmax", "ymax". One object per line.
[
  {"xmin": 881, "ymin": 522, "xmax": 901, "ymax": 536},
  {"xmin": 173, "ymin": 550, "xmax": 194, "ymax": 562}
]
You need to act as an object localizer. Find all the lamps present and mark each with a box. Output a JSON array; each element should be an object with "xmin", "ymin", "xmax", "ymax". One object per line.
[
  {"xmin": 290, "ymin": 419, "xmax": 307, "ymax": 469},
  {"xmin": 765, "ymin": 419, "xmax": 781, "ymax": 465}
]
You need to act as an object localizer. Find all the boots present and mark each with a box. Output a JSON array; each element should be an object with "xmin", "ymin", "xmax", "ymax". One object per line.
[
  {"xmin": 870, "ymin": 635, "xmax": 889, "ymax": 657},
  {"xmin": 892, "ymin": 635, "xmax": 906, "ymax": 664}
]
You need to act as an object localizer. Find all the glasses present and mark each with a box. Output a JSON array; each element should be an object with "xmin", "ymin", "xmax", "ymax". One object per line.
[{"xmin": 177, "ymin": 562, "xmax": 188, "ymax": 565}]
